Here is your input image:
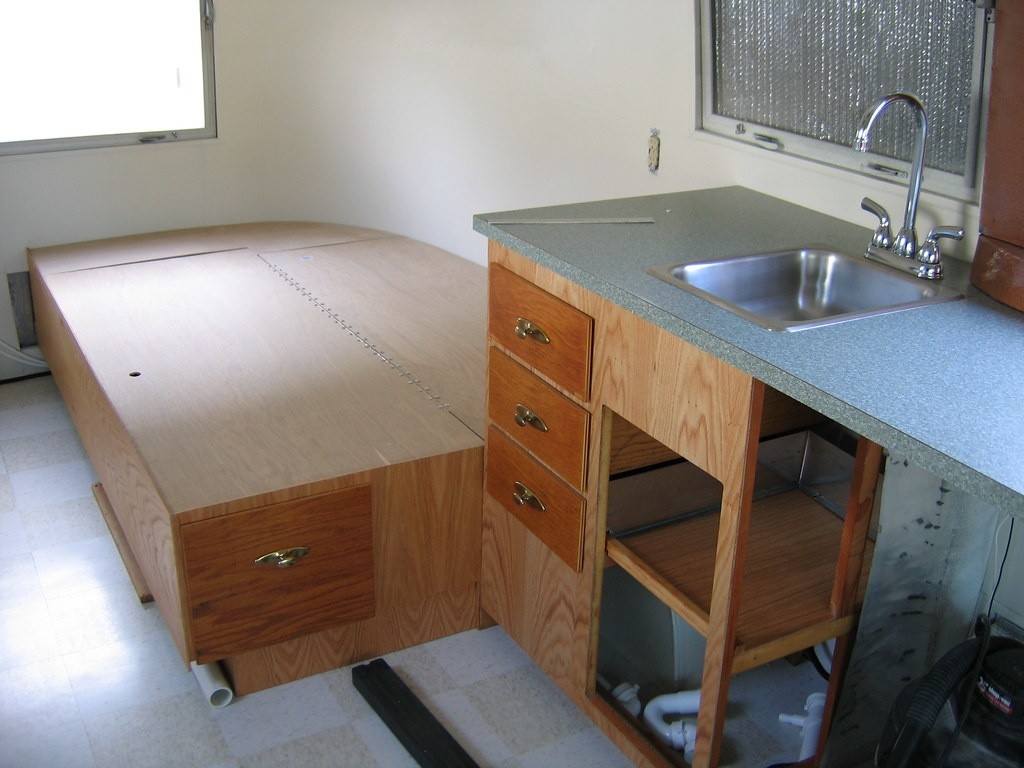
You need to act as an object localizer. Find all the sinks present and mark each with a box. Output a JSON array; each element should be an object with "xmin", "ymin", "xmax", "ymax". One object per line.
[{"xmin": 646, "ymin": 241, "xmax": 965, "ymax": 333}]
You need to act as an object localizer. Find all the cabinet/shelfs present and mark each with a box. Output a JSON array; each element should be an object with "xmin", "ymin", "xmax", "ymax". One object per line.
[{"xmin": 481, "ymin": 237, "xmax": 887, "ymax": 768}]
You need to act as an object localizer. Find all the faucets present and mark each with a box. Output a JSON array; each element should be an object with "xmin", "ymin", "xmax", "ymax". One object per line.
[{"xmin": 850, "ymin": 91, "xmax": 966, "ymax": 283}]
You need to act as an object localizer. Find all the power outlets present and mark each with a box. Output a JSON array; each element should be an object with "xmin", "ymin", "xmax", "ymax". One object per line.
[{"xmin": 647, "ymin": 135, "xmax": 660, "ymax": 171}]
